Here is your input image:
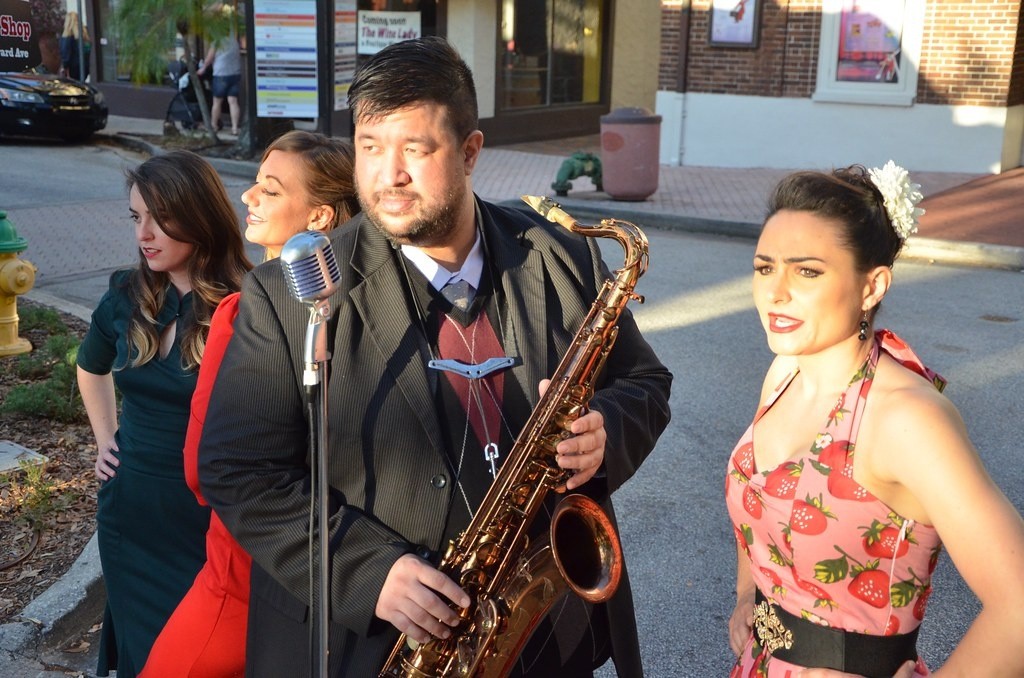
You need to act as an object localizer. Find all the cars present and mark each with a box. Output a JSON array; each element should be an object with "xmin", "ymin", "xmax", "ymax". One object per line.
[{"xmin": 1, "ymin": 69, "xmax": 109, "ymax": 142}]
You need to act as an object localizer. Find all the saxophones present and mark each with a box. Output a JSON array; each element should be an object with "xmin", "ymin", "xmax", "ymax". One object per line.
[{"xmin": 373, "ymin": 195, "xmax": 650, "ymax": 678}]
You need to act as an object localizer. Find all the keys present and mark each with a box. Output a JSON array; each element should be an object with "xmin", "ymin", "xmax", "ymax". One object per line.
[{"xmin": 485, "ymin": 442, "xmax": 499, "ymax": 481}]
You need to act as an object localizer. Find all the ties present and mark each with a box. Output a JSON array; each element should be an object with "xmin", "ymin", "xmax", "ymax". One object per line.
[{"xmin": 441, "ymin": 281, "xmax": 476, "ymax": 312}]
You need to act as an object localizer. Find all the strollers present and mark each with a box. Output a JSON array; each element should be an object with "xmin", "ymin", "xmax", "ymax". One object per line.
[{"xmin": 165, "ymin": 61, "xmax": 223, "ymax": 130}]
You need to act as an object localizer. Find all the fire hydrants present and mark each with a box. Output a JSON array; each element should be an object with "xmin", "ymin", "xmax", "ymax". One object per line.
[{"xmin": 0, "ymin": 210, "xmax": 38, "ymax": 356}]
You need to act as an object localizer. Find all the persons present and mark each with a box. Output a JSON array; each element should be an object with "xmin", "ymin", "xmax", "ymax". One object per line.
[
  {"xmin": 61, "ymin": 11, "xmax": 92, "ymax": 81},
  {"xmin": 725, "ymin": 157, "xmax": 1024, "ymax": 678},
  {"xmin": 197, "ymin": 35, "xmax": 674, "ymax": 678},
  {"xmin": 197, "ymin": 18, "xmax": 243, "ymax": 135},
  {"xmin": 75, "ymin": 150, "xmax": 256, "ymax": 678},
  {"xmin": 136, "ymin": 130, "xmax": 361, "ymax": 678}
]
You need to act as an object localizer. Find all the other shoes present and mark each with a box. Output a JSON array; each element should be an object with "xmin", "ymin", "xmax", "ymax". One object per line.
[
  {"xmin": 205, "ymin": 126, "xmax": 218, "ymax": 133},
  {"xmin": 232, "ymin": 128, "xmax": 241, "ymax": 135}
]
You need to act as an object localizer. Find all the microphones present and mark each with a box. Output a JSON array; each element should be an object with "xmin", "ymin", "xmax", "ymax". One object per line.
[{"xmin": 279, "ymin": 230, "xmax": 342, "ymax": 404}]
